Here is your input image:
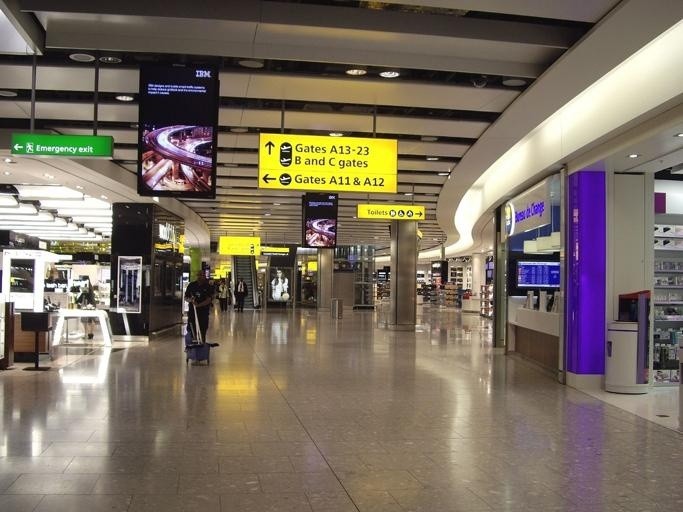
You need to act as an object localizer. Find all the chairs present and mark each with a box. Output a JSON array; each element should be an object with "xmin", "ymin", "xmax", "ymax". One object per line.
[{"xmin": 19, "ymin": 310, "xmax": 52, "ymax": 372}]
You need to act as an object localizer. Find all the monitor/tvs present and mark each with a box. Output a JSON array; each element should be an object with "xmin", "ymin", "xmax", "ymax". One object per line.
[
  {"xmin": 551, "ymin": 292, "xmax": 560, "ymax": 313},
  {"xmin": 525, "ymin": 291, "xmax": 534, "ymax": 310},
  {"xmin": 539, "ymin": 291, "xmax": 547, "ymax": 311}
]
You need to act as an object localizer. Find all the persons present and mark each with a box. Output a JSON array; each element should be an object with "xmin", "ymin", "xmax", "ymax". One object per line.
[
  {"xmin": 81, "ymin": 316, "xmax": 97, "ymax": 340},
  {"xmin": 271, "ymin": 269, "xmax": 288, "ymax": 300},
  {"xmin": 184, "ymin": 269, "xmax": 215, "ymax": 351},
  {"xmin": 208, "ymin": 277, "xmax": 232, "ymax": 313},
  {"xmin": 234, "ymin": 277, "xmax": 248, "ymax": 311},
  {"xmin": 78, "ymin": 275, "xmax": 97, "ymax": 308}
]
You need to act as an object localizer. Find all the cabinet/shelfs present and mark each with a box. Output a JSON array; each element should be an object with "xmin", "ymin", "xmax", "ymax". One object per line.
[
  {"xmin": 370, "ymin": 259, "xmax": 493, "ymax": 320},
  {"xmin": 648, "ymin": 210, "xmax": 682, "ymax": 390}
]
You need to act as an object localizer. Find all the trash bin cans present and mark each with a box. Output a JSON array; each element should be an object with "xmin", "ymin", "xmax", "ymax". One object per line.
[{"xmin": 331, "ymin": 298, "xmax": 343, "ymax": 320}]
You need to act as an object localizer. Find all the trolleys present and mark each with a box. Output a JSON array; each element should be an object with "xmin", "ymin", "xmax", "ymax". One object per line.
[{"xmin": 182, "ymin": 293, "xmax": 209, "ymax": 366}]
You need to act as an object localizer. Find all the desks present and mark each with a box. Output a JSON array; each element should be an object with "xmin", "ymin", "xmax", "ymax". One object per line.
[{"xmin": 51, "ymin": 307, "xmax": 114, "ymax": 347}]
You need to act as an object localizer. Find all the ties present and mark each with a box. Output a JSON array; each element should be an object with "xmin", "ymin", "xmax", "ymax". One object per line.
[{"xmin": 240, "ymin": 284, "xmax": 242, "ymax": 292}]
